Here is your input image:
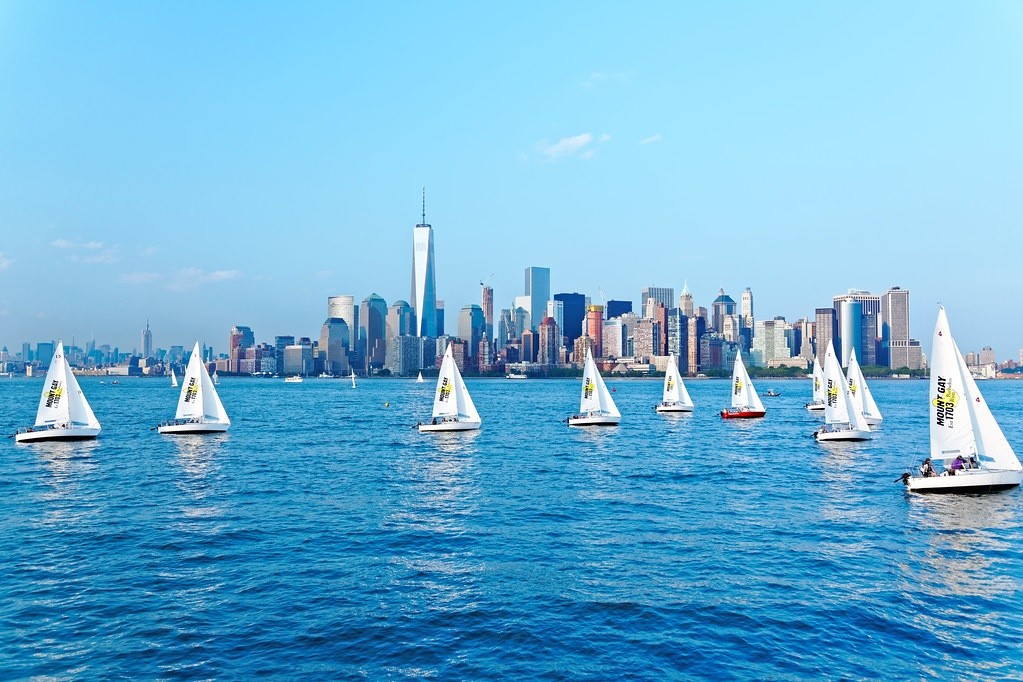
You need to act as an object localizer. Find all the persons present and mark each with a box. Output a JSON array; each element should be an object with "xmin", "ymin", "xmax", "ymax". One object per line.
[
  {"xmin": 819, "ymin": 425, "xmax": 828, "ymax": 433},
  {"xmin": 969, "ymin": 457, "xmax": 979, "ymax": 469},
  {"xmin": 948, "ymin": 455, "xmax": 966, "ymax": 476},
  {"xmin": 174, "ymin": 420, "xmax": 179, "ymax": 425},
  {"xmin": 432, "ymin": 418, "xmax": 437, "ymax": 425},
  {"xmin": 920, "ymin": 458, "xmax": 938, "ymax": 477},
  {"xmin": 166, "ymin": 420, "xmax": 170, "ymax": 426}
]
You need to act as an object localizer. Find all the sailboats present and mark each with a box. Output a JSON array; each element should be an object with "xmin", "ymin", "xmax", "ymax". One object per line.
[
  {"xmin": 150, "ymin": 340, "xmax": 231, "ymax": 434},
  {"xmin": 171, "ymin": 367, "xmax": 178, "ymax": 386},
  {"xmin": 562, "ymin": 345, "xmax": 622, "ymax": 424},
  {"xmin": 810, "ymin": 337, "xmax": 873, "ymax": 441},
  {"xmin": 409, "ymin": 341, "xmax": 481, "ymax": 432},
  {"xmin": 212, "ymin": 369, "xmax": 220, "ymax": 385},
  {"xmin": 415, "ymin": 370, "xmax": 424, "ymax": 383},
  {"xmin": 716, "ymin": 348, "xmax": 767, "ymax": 419},
  {"xmin": 350, "ymin": 367, "xmax": 357, "ymax": 389},
  {"xmin": 651, "ymin": 351, "xmax": 694, "ymax": 412},
  {"xmin": 7, "ymin": 340, "xmax": 103, "ymax": 442},
  {"xmin": 803, "ymin": 353, "xmax": 827, "ymax": 410},
  {"xmin": 893, "ymin": 306, "xmax": 1023, "ymax": 498},
  {"xmin": 846, "ymin": 347, "xmax": 882, "ymax": 425}
]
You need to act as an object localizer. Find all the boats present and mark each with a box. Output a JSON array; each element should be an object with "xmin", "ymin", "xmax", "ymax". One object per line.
[
  {"xmin": 284, "ymin": 373, "xmax": 303, "ymax": 383},
  {"xmin": 972, "ymin": 374, "xmax": 989, "ymax": 380},
  {"xmin": 318, "ymin": 371, "xmax": 333, "ymax": 378},
  {"xmin": 760, "ymin": 388, "xmax": 782, "ymax": 396},
  {"xmin": 505, "ymin": 373, "xmax": 528, "ymax": 379}
]
